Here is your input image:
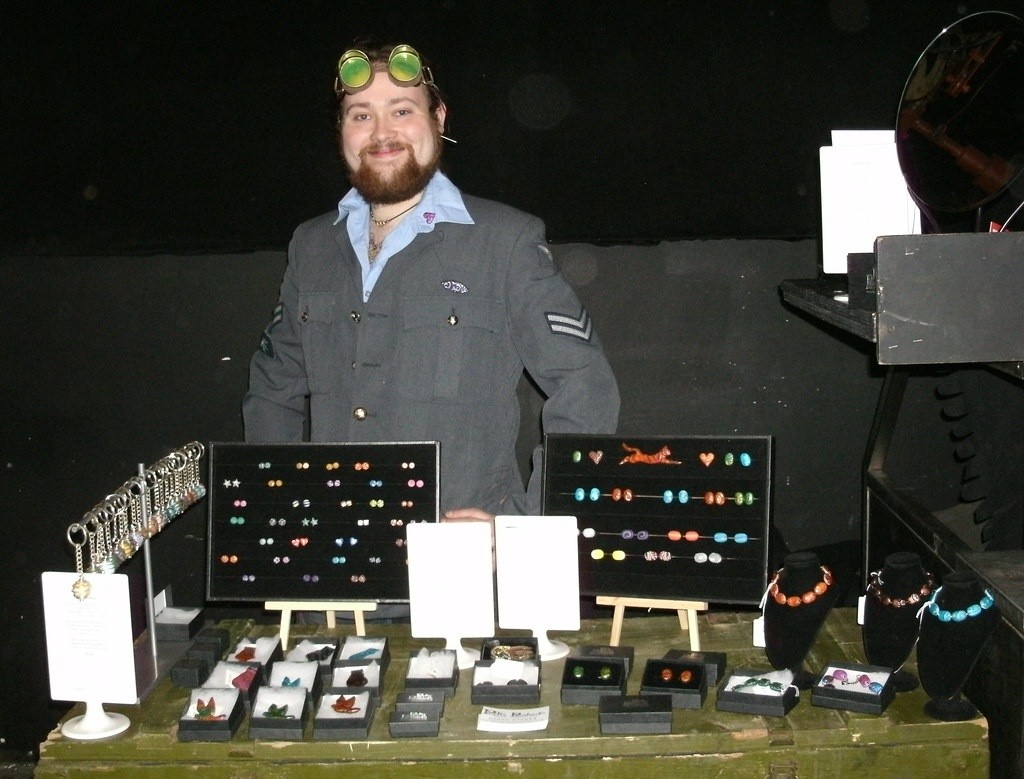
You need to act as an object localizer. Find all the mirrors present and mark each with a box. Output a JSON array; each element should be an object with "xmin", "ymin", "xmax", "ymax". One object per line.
[{"xmin": 895, "ymin": 9, "xmax": 1024, "ymax": 233}]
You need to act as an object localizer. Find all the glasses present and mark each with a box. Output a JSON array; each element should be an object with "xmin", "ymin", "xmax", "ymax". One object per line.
[{"xmin": 333, "ymin": 44, "xmax": 434, "ymax": 95}]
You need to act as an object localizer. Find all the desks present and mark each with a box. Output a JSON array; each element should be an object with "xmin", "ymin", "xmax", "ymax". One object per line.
[
  {"xmin": 778, "ymin": 278, "xmax": 1024, "ymax": 644},
  {"xmin": 31, "ymin": 603, "xmax": 990, "ymax": 779}
]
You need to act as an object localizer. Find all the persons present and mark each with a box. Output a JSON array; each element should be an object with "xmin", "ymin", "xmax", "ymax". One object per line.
[{"xmin": 242, "ymin": 47, "xmax": 624, "ymax": 625}]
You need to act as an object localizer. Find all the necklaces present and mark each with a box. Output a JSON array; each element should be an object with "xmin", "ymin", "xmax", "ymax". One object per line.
[
  {"xmin": 928, "ymin": 581, "xmax": 996, "ymax": 622},
  {"xmin": 758, "ymin": 561, "xmax": 834, "ymax": 608},
  {"xmin": 866, "ymin": 569, "xmax": 936, "ymax": 606}
]
